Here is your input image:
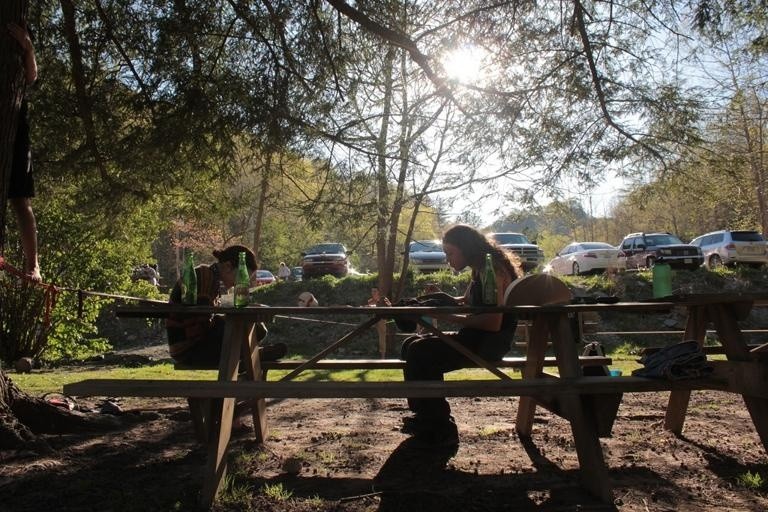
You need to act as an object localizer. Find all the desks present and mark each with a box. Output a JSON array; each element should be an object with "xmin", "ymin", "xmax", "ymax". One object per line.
[{"xmin": 115, "ymin": 291, "xmax": 767, "ymax": 504}]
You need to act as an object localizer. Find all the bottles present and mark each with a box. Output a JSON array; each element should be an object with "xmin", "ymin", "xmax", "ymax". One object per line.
[
  {"xmin": 482, "ymin": 253, "xmax": 498, "ymax": 306},
  {"xmin": 652, "ymin": 256, "xmax": 672, "ymax": 299},
  {"xmin": 234, "ymin": 251, "xmax": 249, "ymax": 308},
  {"xmin": 181, "ymin": 254, "xmax": 197, "ymax": 305}
]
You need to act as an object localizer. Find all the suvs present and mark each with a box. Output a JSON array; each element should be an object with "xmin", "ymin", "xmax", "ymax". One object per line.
[{"xmin": 487, "ymin": 231, "xmax": 545, "ymax": 270}]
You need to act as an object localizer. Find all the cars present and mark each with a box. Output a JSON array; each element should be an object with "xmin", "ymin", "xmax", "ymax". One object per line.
[
  {"xmin": 400, "ymin": 240, "xmax": 450, "ymax": 274},
  {"xmin": 290, "ymin": 267, "xmax": 302, "ymax": 283},
  {"xmin": 300, "ymin": 242, "xmax": 352, "ymax": 279},
  {"xmin": 619, "ymin": 231, "xmax": 704, "ymax": 270},
  {"xmin": 547, "ymin": 240, "xmax": 627, "ymax": 278},
  {"xmin": 250, "ymin": 267, "xmax": 275, "ymax": 289},
  {"xmin": 689, "ymin": 228, "xmax": 767, "ymax": 271}
]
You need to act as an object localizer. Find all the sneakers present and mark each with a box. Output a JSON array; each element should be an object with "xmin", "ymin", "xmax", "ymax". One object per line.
[
  {"xmin": 402, "ymin": 413, "xmax": 459, "ymax": 451},
  {"xmin": 216, "ymin": 414, "xmax": 252, "ymax": 436}
]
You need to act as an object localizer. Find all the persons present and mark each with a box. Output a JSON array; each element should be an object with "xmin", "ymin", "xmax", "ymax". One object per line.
[
  {"xmin": 1, "ymin": 23, "xmax": 44, "ymax": 284},
  {"xmin": 167, "ymin": 244, "xmax": 288, "ymax": 436},
  {"xmin": 277, "ymin": 262, "xmax": 290, "ymax": 282},
  {"xmin": 402, "ymin": 224, "xmax": 522, "ymax": 450},
  {"xmin": 134, "ymin": 262, "xmax": 162, "ymax": 289},
  {"xmin": 367, "ymin": 287, "xmax": 393, "ymax": 358}
]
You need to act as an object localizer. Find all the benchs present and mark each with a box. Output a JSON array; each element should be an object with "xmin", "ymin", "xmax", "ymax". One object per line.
[{"xmin": 47, "ymin": 354, "xmax": 767, "ymax": 512}]
